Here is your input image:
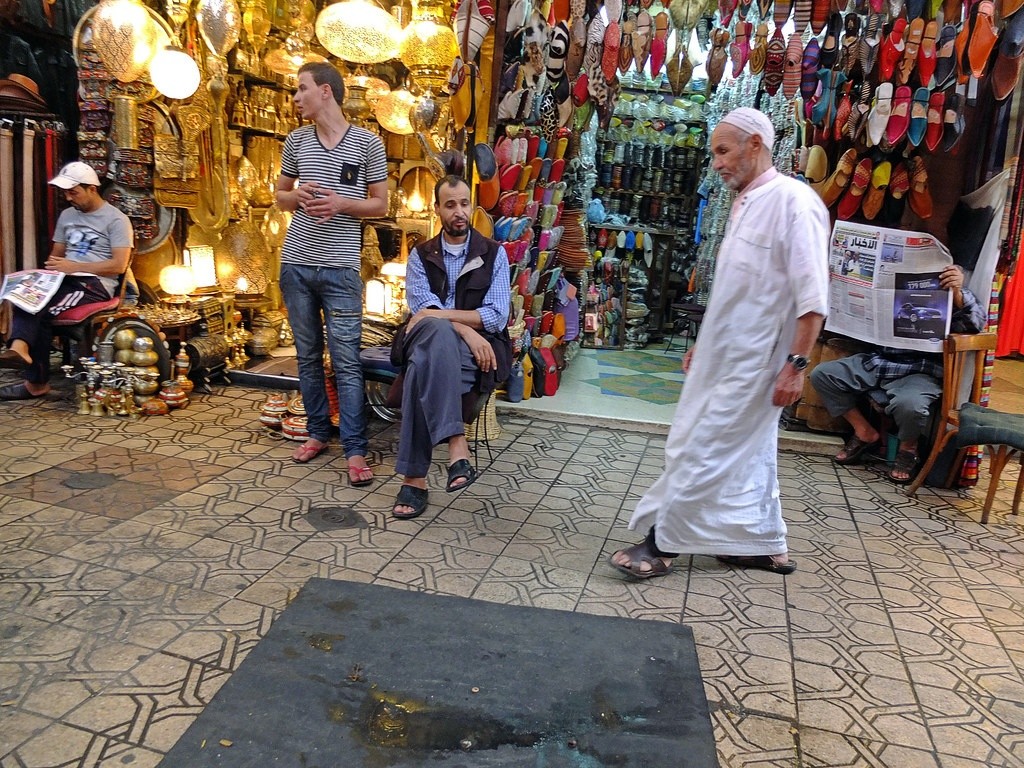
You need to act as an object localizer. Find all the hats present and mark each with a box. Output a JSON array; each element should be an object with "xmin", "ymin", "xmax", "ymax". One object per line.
[
  {"xmin": 47, "ymin": 162, "xmax": 100, "ymax": 189},
  {"xmin": 719, "ymin": 107, "xmax": 775, "ymax": 150}
]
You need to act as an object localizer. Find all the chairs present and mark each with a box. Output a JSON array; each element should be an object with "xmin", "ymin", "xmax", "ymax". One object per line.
[
  {"xmin": 906, "ymin": 333, "xmax": 1024, "ymax": 525},
  {"xmin": 52, "ymin": 249, "xmax": 137, "ymax": 370}
]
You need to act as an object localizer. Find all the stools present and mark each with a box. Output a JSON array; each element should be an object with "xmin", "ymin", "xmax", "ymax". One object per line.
[{"xmin": 664, "ymin": 304, "xmax": 705, "ymax": 354}]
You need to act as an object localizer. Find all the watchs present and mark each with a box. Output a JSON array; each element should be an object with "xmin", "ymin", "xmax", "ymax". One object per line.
[{"xmin": 788, "ymin": 354, "xmax": 812, "ymax": 370}]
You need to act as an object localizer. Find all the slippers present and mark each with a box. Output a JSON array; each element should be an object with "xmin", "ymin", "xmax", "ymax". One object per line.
[
  {"xmin": 392, "ymin": 485, "xmax": 428, "ymax": 518},
  {"xmin": 292, "ymin": 444, "xmax": 328, "ymax": 464},
  {"xmin": 348, "ymin": 465, "xmax": 374, "ymax": 487},
  {"xmin": 446, "ymin": 458, "xmax": 474, "ymax": 493},
  {"xmin": 0, "ymin": 384, "xmax": 50, "ymax": 400},
  {"xmin": 447, "ymin": 0, "xmax": 1023, "ymax": 403},
  {"xmin": 607, "ymin": 542, "xmax": 674, "ymax": 579},
  {"xmin": 833, "ymin": 436, "xmax": 879, "ymax": 465},
  {"xmin": 716, "ymin": 554, "xmax": 798, "ymax": 575},
  {"xmin": 889, "ymin": 447, "xmax": 920, "ymax": 483},
  {"xmin": 0, "ymin": 351, "xmax": 31, "ymax": 368}
]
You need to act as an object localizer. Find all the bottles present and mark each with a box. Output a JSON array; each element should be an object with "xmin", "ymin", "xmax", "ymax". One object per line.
[{"xmin": 343, "ymin": 85, "xmax": 372, "ymax": 128}]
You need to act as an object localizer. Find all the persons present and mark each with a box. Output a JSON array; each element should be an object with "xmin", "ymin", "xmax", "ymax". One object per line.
[
  {"xmin": 0, "ymin": 161, "xmax": 134, "ymax": 400},
  {"xmin": 606, "ymin": 107, "xmax": 831, "ymax": 578},
  {"xmin": 807, "ymin": 230, "xmax": 987, "ymax": 485},
  {"xmin": 273, "ymin": 61, "xmax": 388, "ymax": 487},
  {"xmin": 391, "ymin": 175, "xmax": 511, "ymax": 520}
]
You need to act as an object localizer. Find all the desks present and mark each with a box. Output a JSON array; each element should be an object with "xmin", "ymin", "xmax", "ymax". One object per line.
[{"xmin": 160, "ymin": 315, "xmax": 203, "ymax": 342}]
